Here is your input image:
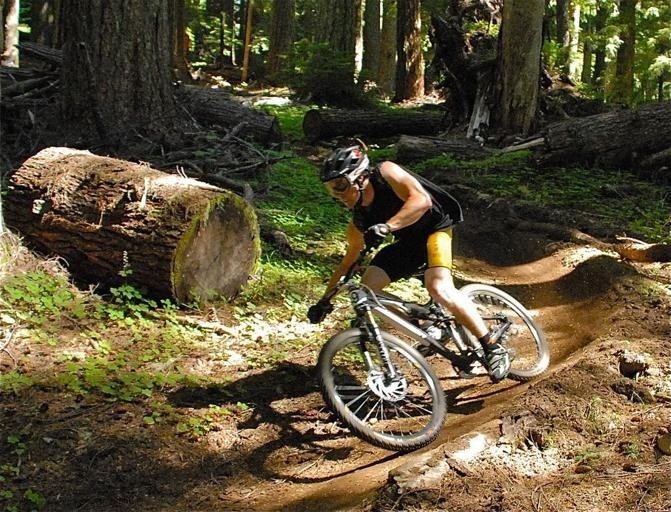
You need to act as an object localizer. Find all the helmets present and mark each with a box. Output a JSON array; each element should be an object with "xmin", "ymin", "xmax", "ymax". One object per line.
[{"xmin": 320, "ymin": 145, "xmax": 370, "ymax": 191}]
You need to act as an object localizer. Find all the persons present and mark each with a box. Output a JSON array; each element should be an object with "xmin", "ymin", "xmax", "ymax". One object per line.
[{"xmin": 307, "ymin": 145, "xmax": 511, "ymax": 382}]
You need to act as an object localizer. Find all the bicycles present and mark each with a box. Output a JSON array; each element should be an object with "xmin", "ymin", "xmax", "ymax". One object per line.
[{"xmin": 307, "ymin": 226, "xmax": 550, "ymax": 451}]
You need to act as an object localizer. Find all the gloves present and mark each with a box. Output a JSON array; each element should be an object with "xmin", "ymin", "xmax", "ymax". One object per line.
[
  {"xmin": 306, "ymin": 298, "xmax": 334, "ymax": 324},
  {"xmin": 363, "ymin": 223, "xmax": 391, "ymax": 249}
]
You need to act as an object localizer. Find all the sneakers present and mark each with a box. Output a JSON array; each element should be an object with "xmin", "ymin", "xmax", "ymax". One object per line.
[
  {"xmin": 483, "ymin": 341, "xmax": 512, "ymax": 384},
  {"xmin": 417, "ymin": 329, "xmax": 451, "ymax": 358}
]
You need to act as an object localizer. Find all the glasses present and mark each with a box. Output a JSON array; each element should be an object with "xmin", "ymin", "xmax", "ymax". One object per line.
[{"xmin": 331, "ymin": 182, "xmax": 350, "ymax": 193}]
